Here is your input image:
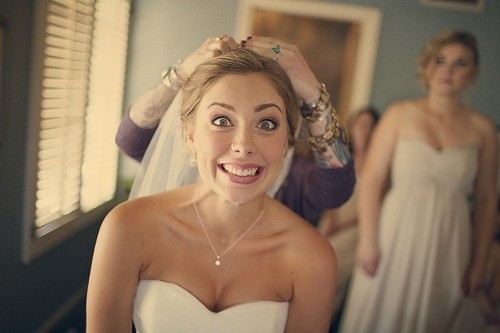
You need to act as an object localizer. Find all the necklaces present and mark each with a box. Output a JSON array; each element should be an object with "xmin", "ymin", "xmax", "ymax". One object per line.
[{"xmin": 193, "ymin": 182, "xmax": 267, "ymax": 266}]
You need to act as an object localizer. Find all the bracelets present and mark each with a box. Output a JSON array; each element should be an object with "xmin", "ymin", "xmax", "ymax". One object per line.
[
  {"xmin": 170, "ymin": 64, "xmax": 187, "ymax": 85},
  {"xmin": 302, "ymin": 82, "xmax": 337, "ymax": 143}
]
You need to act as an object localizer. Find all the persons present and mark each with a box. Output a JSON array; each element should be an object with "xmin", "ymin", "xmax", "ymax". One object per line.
[
  {"xmin": 113, "ymin": 34, "xmax": 356, "ymax": 227},
  {"xmin": 338, "ymin": 29, "xmax": 499, "ymax": 333},
  {"xmin": 85, "ymin": 49, "xmax": 338, "ymax": 333},
  {"xmin": 317, "ymin": 107, "xmax": 381, "ymax": 234}
]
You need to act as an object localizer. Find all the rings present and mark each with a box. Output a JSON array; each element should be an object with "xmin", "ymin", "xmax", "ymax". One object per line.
[
  {"xmin": 271, "ymin": 42, "xmax": 281, "ymax": 53},
  {"xmin": 272, "ymin": 53, "xmax": 280, "ymax": 62},
  {"xmin": 219, "ymin": 34, "xmax": 227, "ymax": 41}
]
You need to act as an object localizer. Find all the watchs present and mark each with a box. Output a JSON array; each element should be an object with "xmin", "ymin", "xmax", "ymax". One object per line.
[{"xmin": 161, "ymin": 67, "xmax": 178, "ymax": 92}]
